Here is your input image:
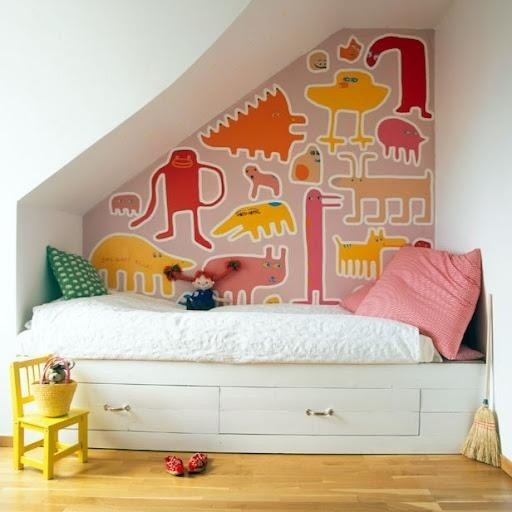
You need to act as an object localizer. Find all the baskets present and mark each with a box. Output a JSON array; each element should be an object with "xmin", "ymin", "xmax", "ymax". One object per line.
[{"xmin": 29, "ymin": 357, "xmax": 77, "ymax": 418}]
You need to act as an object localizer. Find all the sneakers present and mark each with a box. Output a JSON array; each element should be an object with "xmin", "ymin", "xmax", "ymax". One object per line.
[
  {"xmin": 165, "ymin": 455, "xmax": 184, "ymax": 476},
  {"xmin": 188, "ymin": 452, "xmax": 208, "ymax": 473}
]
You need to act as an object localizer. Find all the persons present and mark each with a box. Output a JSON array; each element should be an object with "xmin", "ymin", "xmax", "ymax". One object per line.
[{"xmin": 163, "ymin": 260, "xmax": 241, "ymax": 310}]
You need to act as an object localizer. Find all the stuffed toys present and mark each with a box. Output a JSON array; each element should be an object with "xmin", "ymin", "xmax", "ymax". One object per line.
[{"xmin": 43, "ymin": 363, "xmax": 65, "ymax": 384}]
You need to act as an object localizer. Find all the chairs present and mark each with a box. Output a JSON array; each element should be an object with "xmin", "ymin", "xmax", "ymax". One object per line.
[{"xmin": 9, "ymin": 354, "xmax": 89, "ymax": 479}]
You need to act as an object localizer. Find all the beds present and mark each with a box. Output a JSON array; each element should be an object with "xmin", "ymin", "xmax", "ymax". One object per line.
[{"xmin": 17, "ymin": 290, "xmax": 488, "ymax": 455}]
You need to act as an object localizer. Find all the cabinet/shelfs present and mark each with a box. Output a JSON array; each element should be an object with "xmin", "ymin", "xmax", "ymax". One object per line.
[{"xmin": 19, "ymin": 362, "xmax": 487, "ymax": 456}]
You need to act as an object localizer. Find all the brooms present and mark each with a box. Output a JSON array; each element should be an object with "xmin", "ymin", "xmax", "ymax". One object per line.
[{"xmin": 459, "ymin": 294, "xmax": 500, "ymax": 467}]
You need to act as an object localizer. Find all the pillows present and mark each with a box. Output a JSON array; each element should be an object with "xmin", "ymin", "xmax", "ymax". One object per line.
[
  {"xmin": 46, "ymin": 245, "xmax": 112, "ymax": 301},
  {"xmin": 338, "ymin": 243, "xmax": 482, "ymax": 360}
]
